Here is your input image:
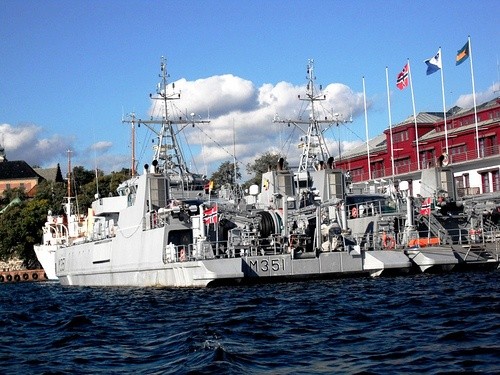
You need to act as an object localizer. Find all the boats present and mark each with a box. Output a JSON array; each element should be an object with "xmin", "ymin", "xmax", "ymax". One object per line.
[
  {"xmin": 269, "ymin": 58, "xmax": 354, "ymax": 199},
  {"xmin": 32, "ymin": 57, "xmax": 500, "ymax": 290}
]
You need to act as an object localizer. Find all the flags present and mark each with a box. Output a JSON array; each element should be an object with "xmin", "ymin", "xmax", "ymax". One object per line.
[
  {"xmin": 396, "ymin": 63, "xmax": 409, "ymax": 89},
  {"xmin": 421, "ymin": 198, "xmax": 430, "ymax": 214},
  {"xmin": 202, "ymin": 205, "xmax": 217, "ymax": 226},
  {"xmin": 202, "ymin": 179, "xmax": 214, "ymax": 194},
  {"xmin": 455, "ymin": 42, "xmax": 469, "ymax": 66},
  {"xmin": 424, "ymin": 49, "xmax": 442, "ymax": 75}
]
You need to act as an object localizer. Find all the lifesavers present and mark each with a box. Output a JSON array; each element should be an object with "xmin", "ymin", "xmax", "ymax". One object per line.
[
  {"xmin": 351, "ymin": 207, "xmax": 358, "ymax": 218},
  {"xmin": 180, "ymin": 249, "xmax": 186, "ymax": 262},
  {"xmin": 383, "ymin": 236, "xmax": 395, "ymax": 250}
]
